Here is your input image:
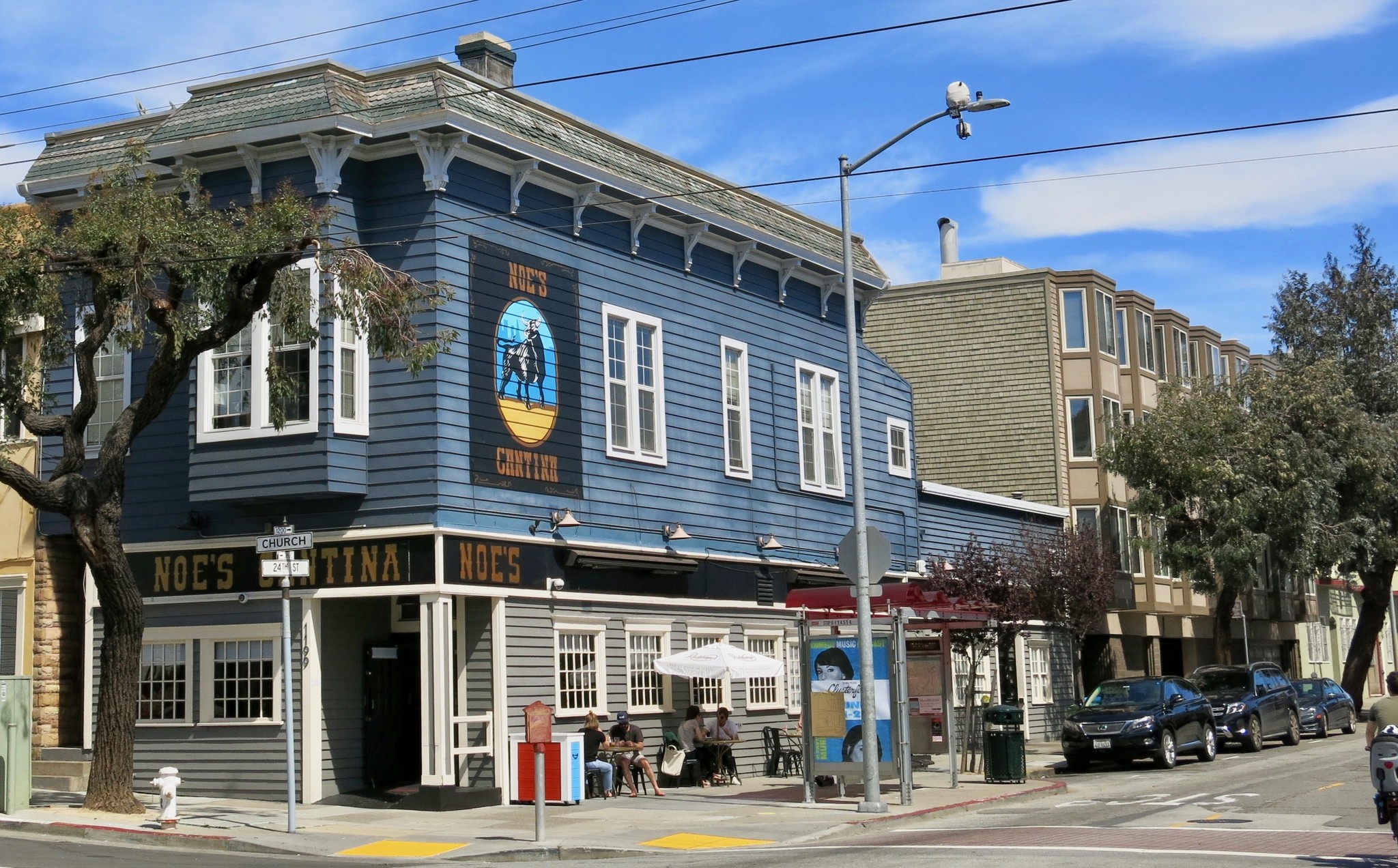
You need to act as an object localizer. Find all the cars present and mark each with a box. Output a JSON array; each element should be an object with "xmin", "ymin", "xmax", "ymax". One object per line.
[
  {"xmin": 1060, "ymin": 674, "xmax": 1220, "ymax": 772},
  {"xmin": 1287, "ymin": 675, "xmax": 1357, "ymax": 739}
]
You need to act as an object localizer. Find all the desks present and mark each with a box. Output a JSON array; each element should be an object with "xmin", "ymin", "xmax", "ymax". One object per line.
[
  {"xmin": 702, "ymin": 739, "xmax": 745, "ymax": 786},
  {"xmin": 598, "ymin": 745, "xmax": 637, "ymax": 797},
  {"xmin": 778, "ymin": 733, "xmax": 802, "ymax": 775}
]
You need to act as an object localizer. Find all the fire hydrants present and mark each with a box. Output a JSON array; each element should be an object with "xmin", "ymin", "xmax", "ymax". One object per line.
[{"xmin": 149, "ymin": 766, "xmax": 186, "ymax": 829}]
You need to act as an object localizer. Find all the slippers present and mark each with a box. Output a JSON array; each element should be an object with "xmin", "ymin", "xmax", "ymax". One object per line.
[
  {"xmin": 630, "ymin": 793, "xmax": 637, "ymax": 797},
  {"xmin": 655, "ymin": 792, "xmax": 665, "ymax": 796}
]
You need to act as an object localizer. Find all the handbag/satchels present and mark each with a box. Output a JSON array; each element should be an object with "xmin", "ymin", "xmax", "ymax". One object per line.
[{"xmin": 661, "ymin": 744, "xmax": 686, "ymax": 776}]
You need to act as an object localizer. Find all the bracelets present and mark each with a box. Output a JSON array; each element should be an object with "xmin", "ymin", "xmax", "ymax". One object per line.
[
  {"xmin": 1367, "ymin": 746, "xmax": 1371, "ymax": 748},
  {"xmin": 610, "ymin": 743, "xmax": 611, "ymax": 747}
]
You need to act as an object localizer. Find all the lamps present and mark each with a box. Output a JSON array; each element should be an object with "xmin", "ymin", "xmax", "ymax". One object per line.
[
  {"xmin": 756, "ymin": 533, "xmax": 784, "ymax": 550},
  {"xmin": 530, "ymin": 508, "xmax": 582, "ymax": 536},
  {"xmin": 663, "ymin": 521, "xmax": 691, "ymax": 542}
]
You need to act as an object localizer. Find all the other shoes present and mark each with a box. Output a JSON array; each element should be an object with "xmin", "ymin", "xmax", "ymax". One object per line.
[
  {"xmin": 713, "ymin": 774, "xmax": 726, "ymax": 781},
  {"xmin": 701, "ymin": 779, "xmax": 711, "ymax": 787},
  {"xmin": 731, "ymin": 776, "xmax": 741, "ymax": 785},
  {"xmin": 602, "ymin": 790, "xmax": 614, "ymax": 797}
]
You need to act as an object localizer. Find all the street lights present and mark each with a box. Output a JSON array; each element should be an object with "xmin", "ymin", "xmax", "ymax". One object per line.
[
  {"xmin": 1231, "ymin": 600, "xmax": 1250, "ymax": 666},
  {"xmin": 837, "ymin": 94, "xmax": 1010, "ymax": 812}
]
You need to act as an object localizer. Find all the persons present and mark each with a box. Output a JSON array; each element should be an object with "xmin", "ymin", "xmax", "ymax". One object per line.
[
  {"xmin": 1311, "ymin": 672, "xmax": 1319, "ymax": 689},
  {"xmin": 814, "ymin": 647, "xmax": 854, "ymax": 681},
  {"xmin": 701, "ymin": 707, "xmax": 741, "ymax": 785},
  {"xmin": 842, "ymin": 725, "xmax": 883, "ymax": 762},
  {"xmin": 1365, "ymin": 671, "xmax": 1398, "ymax": 750},
  {"xmin": 606, "ymin": 711, "xmax": 665, "ymax": 797},
  {"xmin": 677, "ymin": 705, "xmax": 726, "ymax": 787},
  {"xmin": 578, "ymin": 711, "xmax": 618, "ymax": 797}
]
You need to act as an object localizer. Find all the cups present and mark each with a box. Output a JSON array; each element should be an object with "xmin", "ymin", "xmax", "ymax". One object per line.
[
  {"xmin": 614, "ymin": 737, "xmax": 620, "ymax": 748},
  {"xmin": 782, "ymin": 728, "xmax": 787, "ymax": 735},
  {"xmin": 608, "ymin": 739, "xmax": 611, "ymax": 747},
  {"xmin": 796, "ymin": 728, "xmax": 801, "ymax": 732}
]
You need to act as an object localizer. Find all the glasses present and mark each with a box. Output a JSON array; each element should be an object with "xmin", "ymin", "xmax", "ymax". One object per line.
[
  {"xmin": 719, "ymin": 716, "xmax": 726, "ymax": 719},
  {"xmin": 619, "ymin": 721, "xmax": 629, "ymax": 725}
]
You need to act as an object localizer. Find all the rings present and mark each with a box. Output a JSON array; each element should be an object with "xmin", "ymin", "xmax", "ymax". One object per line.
[{"xmin": 630, "ymin": 743, "xmax": 631, "ymax": 745}]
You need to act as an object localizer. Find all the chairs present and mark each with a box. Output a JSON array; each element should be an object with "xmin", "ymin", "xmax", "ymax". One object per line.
[
  {"xmin": 763, "ymin": 726, "xmax": 805, "ymax": 780},
  {"xmin": 583, "ymin": 759, "xmax": 607, "ymax": 801},
  {"xmin": 664, "ymin": 730, "xmax": 705, "ymax": 789},
  {"xmin": 612, "ymin": 751, "xmax": 648, "ymax": 796}
]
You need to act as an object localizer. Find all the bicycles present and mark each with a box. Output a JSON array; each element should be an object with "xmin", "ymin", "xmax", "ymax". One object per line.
[{"xmin": 1365, "ymin": 730, "xmax": 1398, "ymax": 842}]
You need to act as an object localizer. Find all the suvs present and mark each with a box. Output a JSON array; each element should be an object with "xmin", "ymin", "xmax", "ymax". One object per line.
[{"xmin": 1187, "ymin": 661, "xmax": 1302, "ymax": 752}]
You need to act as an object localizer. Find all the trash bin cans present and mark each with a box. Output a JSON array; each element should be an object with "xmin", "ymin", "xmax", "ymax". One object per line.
[{"xmin": 982, "ymin": 703, "xmax": 1028, "ymax": 784}]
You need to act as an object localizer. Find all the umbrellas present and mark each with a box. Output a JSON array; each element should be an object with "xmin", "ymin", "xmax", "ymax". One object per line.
[
  {"xmin": 653, "ymin": 639, "xmax": 785, "ymax": 786},
  {"xmin": 570, "ymin": 652, "xmax": 655, "ymax": 686}
]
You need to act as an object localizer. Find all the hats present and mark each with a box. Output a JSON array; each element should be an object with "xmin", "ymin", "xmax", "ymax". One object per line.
[{"xmin": 617, "ymin": 712, "xmax": 629, "ymax": 723}]
